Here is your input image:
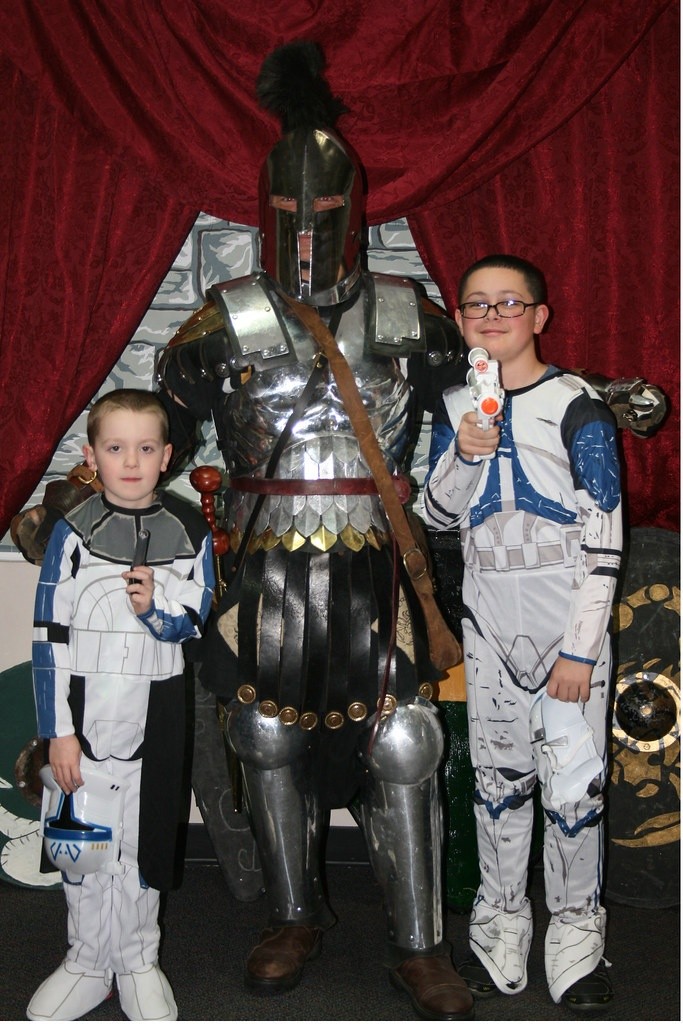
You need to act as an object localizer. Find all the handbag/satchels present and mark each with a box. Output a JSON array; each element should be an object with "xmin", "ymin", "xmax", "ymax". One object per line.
[{"xmin": 403, "ymin": 511, "xmax": 465, "ymax": 670}]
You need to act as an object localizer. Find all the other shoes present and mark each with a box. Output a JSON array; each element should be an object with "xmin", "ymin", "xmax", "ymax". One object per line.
[
  {"xmin": 562, "ymin": 958, "xmax": 613, "ymax": 1011},
  {"xmin": 25, "ymin": 963, "xmax": 114, "ymax": 1020},
  {"xmin": 451, "ymin": 933, "xmax": 502, "ymax": 999},
  {"xmin": 117, "ymin": 967, "xmax": 178, "ymax": 1021}
]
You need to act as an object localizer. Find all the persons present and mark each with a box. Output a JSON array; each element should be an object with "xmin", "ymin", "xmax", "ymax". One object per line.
[
  {"xmin": 12, "ymin": 35, "xmax": 669, "ymax": 1021},
  {"xmin": 425, "ymin": 255, "xmax": 625, "ymax": 1010},
  {"xmin": 24, "ymin": 388, "xmax": 216, "ymax": 1021}
]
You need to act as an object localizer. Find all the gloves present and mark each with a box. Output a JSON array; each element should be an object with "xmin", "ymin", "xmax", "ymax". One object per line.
[
  {"xmin": 582, "ymin": 374, "xmax": 668, "ymax": 439},
  {"xmin": 11, "ymin": 480, "xmax": 84, "ymax": 567}
]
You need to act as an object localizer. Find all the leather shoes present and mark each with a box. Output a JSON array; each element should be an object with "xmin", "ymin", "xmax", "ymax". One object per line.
[
  {"xmin": 243, "ymin": 925, "xmax": 323, "ymax": 991},
  {"xmin": 392, "ymin": 954, "xmax": 473, "ymax": 1021}
]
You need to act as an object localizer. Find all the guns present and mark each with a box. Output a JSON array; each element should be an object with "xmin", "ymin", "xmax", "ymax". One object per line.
[
  {"xmin": 130, "ymin": 529, "xmax": 151, "ymax": 595},
  {"xmin": 466, "ymin": 347, "xmax": 506, "ymax": 460}
]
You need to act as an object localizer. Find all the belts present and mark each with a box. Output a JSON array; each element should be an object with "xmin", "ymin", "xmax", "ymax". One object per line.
[{"xmin": 231, "ymin": 477, "xmax": 379, "ymax": 495}]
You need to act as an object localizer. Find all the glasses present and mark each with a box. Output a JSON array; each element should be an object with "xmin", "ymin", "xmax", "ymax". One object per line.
[{"xmin": 459, "ymin": 300, "xmax": 540, "ymax": 319}]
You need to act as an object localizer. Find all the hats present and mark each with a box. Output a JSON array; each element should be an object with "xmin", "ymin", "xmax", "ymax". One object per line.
[{"xmin": 256, "ymin": 39, "xmax": 363, "ymax": 301}]
